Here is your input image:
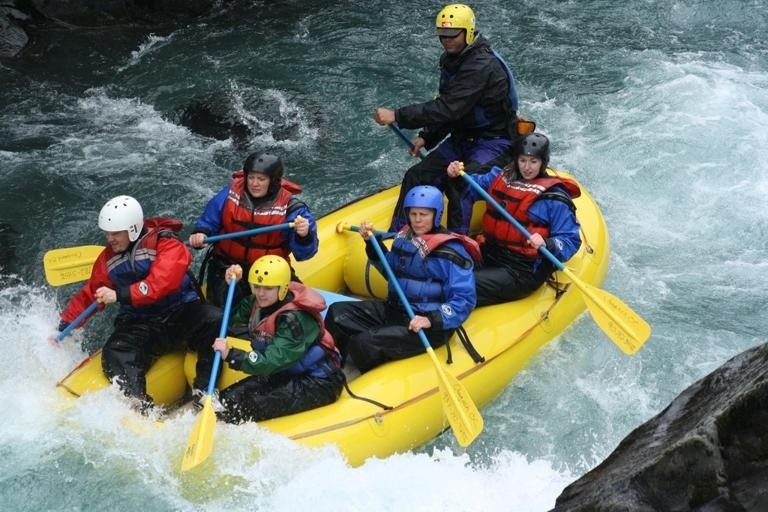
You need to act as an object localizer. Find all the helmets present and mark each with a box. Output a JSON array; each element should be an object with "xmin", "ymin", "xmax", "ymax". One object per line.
[
  {"xmin": 432, "ymin": 5, "xmax": 475, "ymax": 46},
  {"xmin": 514, "ymin": 133, "xmax": 549, "ymax": 176},
  {"xmin": 405, "ymin": 186, "xmax": 443, "ymax": 228},
  {"xmin": 248, "ymin": 255, "xmax": 292, "ymax": 302},
  {"xmin": 243, "ymin": 153, "xmax": 284, "ymax": 191},
  {"xmin": 97, "ymin": 193, "xmax": 144, "ymax": 242}
]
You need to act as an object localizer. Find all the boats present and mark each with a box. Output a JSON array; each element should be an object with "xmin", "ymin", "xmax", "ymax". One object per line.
[{"xmin": 55, "ymin": 167, "xmax": 611, "ymax": 500}]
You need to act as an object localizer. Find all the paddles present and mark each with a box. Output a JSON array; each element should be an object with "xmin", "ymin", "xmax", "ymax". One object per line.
[
  {"xmin": 43, "ymin": 214, "xmax": 303, "ymax": 286},
  {"xmin": 181, "ymin": 273, "xmax": 236, "ymax": 472},
  {"xmin": 459, "ymin": 167, "xmax": 651, "ymax": 354},
  {"xmin": 367, "ymin": 228, "xmax": 485, "ymax": 447}
]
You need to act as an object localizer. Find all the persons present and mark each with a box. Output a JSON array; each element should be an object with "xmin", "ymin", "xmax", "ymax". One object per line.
[
  {"xmin": 48, "ymin": 193, "xmax": 223, "ymax": 416},
  {"xmin": 447, "ymin": 131, "xmax": 583, "ymax": 308},
  {"xmin": 372, "ymin": 2, "xmax": 522, "ymax": 236},
  {"xmin": 323, "ymin": 183, "xmax": 478, "ymax": 377},
  {"xmin": 185, "ymin": 153, "xmax": 320, "ymax": 322},
  {"xmin": 211, "ymin": 253, "xmax": 345, "ymax": 425}
]
